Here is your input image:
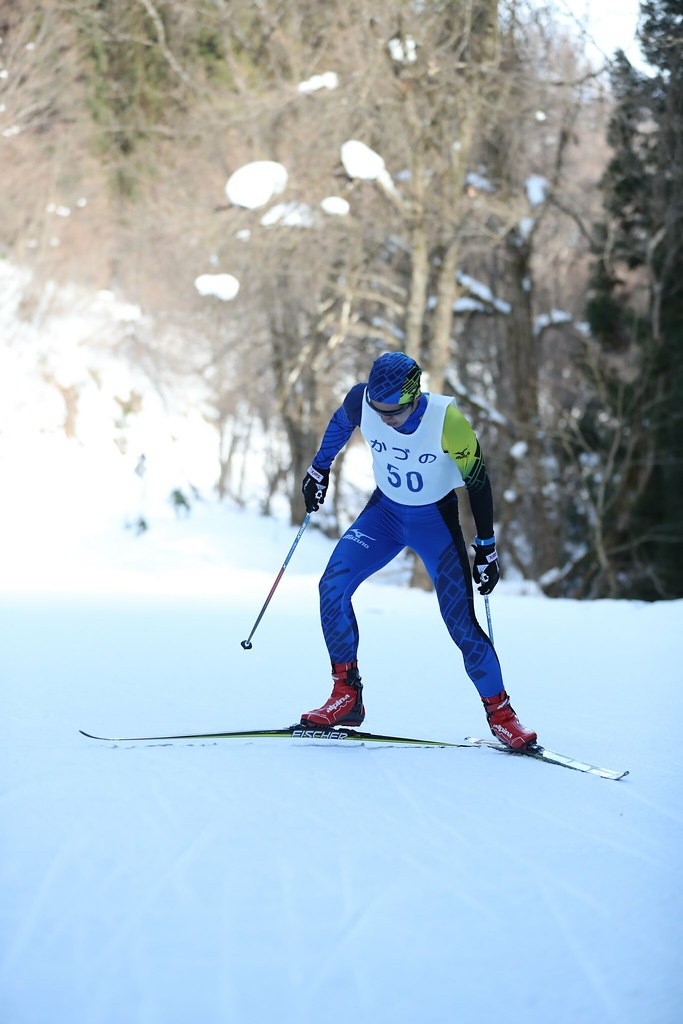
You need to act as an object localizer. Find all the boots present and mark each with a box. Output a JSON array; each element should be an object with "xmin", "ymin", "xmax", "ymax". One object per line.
[
  {"xmin": 301, "ymin": 661, "xmax": 365, "ymax": 728},
  {"xmin": 481, "ymin": 690, "xmax": 536, "ymax": 750}
]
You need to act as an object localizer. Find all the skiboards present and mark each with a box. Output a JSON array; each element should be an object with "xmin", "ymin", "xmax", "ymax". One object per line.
[{"xmin": 77, "ymin": 724, "xmax": 632, "ymax": 783}]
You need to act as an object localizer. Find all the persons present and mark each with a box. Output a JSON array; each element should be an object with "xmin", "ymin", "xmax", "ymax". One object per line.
[{"xmin": 300, "ymin": 353, "xmax": 538, "ymax": 749}]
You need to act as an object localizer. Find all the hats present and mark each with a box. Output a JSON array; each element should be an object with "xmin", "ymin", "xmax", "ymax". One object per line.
[{"xmin": 369, "ymin": 352, "xmax": 422, "ymax": 404}]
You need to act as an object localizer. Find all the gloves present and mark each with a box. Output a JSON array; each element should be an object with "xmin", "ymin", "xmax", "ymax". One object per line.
[
  {"xmin": 470, "ymin": 544, "xmax": 500, "ymax": 595},
  {"xmin": 301, "ymin": 465, "xmax": 331, "ymax": 513}
]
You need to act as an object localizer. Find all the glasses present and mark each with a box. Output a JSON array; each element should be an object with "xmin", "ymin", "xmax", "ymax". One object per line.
[{"xmin": 366, "ymin": 396, "xmax": 409, "ymax": 415}]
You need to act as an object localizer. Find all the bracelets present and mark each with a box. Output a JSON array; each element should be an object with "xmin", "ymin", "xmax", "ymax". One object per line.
[{"xmin": 474, "ymin": 535, "xmax": 495, "ymax": 546}]
